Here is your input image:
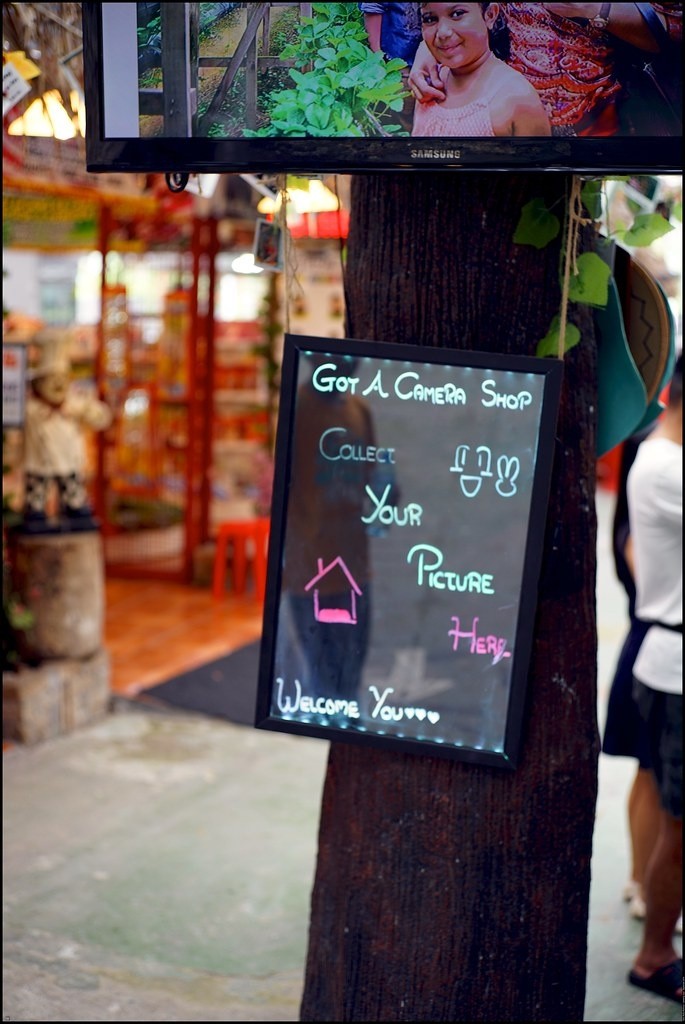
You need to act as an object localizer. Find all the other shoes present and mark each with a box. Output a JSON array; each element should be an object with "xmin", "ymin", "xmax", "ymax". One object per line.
[{"xmin": 622, "ymin": 880, "xmax": 682, "ymax": 935}]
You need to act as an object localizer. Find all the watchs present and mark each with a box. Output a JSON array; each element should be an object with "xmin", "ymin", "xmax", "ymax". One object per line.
[{"xmin": 589, "ymin": 0, "xmax": 611, "ymax": 30}]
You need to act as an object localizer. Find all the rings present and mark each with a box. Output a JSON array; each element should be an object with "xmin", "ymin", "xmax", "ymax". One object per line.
[{"xmin": 410, "ymin": 89, "xmax": 418, "ymax": 97}]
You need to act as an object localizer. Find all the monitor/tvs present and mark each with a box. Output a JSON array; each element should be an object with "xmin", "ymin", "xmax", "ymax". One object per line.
[{"xmin": 78, "ymin": 2, "xmax": 683, "ymax": 178}]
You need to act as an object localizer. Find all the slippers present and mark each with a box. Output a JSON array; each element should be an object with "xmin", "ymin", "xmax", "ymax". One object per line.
[{"xmin": 628, "ymin": 958, "xmax": 683, "ymax": 1004}]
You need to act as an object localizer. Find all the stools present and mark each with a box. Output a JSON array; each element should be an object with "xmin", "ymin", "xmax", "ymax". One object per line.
[{"xmin": 210, "ymin": 520, "xmax": 267, "ymax": 601}]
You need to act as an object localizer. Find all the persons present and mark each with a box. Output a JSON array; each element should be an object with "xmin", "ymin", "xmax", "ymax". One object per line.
[
  {"xmin": 627, "ymin": 353, "xmax": 685, "ymax": 1008},
  {"xmin": 486, "ymin": 0, "xmax": 683, "ymax": 136},
  {"xmin": 359, "ymin": 1, "xmax": 421, "ymax": 68},
  {"xmin": 405, "ymin": 3, "xmax": 553, "ymax": 137},
  {"xmin": 600, "ymin": 425, "xmax": 684, "ymax": 936},
  {"xmin": 21, "ymin": 327, "xmax": 113, "ymax": 523}
]
